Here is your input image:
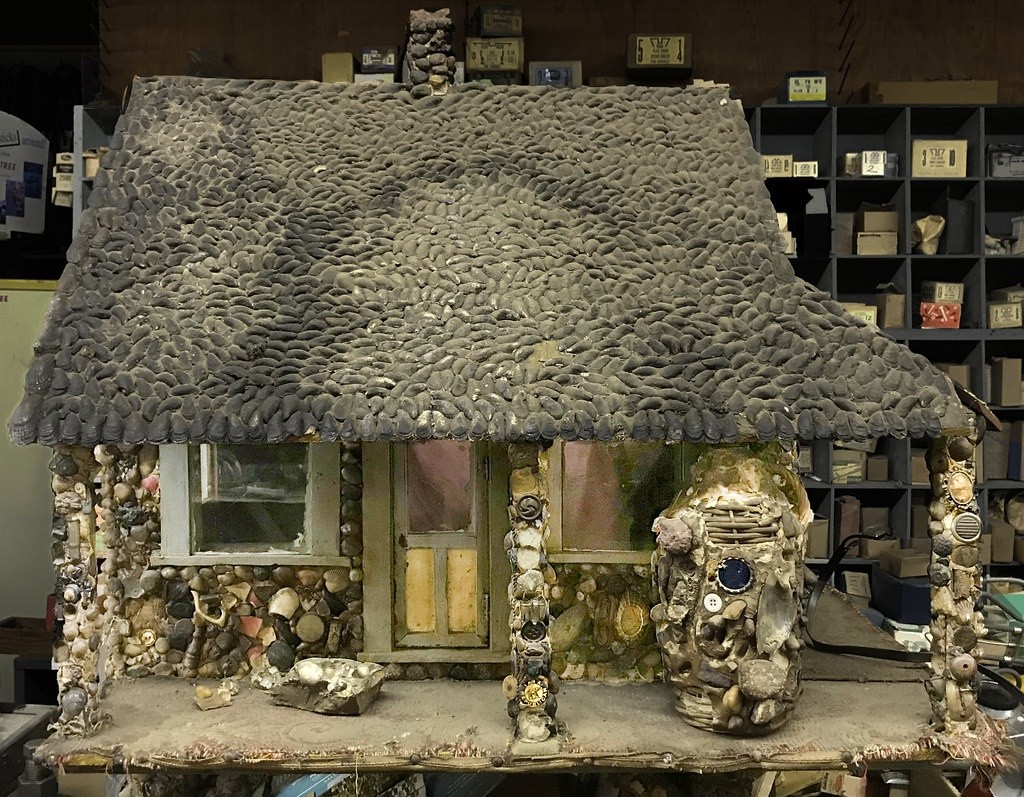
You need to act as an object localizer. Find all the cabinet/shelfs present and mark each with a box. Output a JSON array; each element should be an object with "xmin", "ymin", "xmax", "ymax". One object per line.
[{"xmin": 746, "ymin": 95, "xmax": 1024, "ymax": 619}]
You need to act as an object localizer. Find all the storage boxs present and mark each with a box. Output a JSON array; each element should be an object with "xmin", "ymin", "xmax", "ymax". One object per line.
[
  {"xmin": 780, "ymin": 77, "xmax": 828, "ymax": 103},
  {"xmin": 756, "ymin": 137, "xmax": 1024, "ymax": 178},
  {"xmin": 867, "ymin": 79, "xmax": 1000, "ymax": 105},
  {"xmin": 317, "ymin": 3, "xmax": 694, "ymax": 89},
  {"xmin": 774, "ymin": 196, "xmax": 1023, "ymax": 329},
  {"xmin": 797, "ymin": 356, "xmax": 1024, "ymax": 629}
]
